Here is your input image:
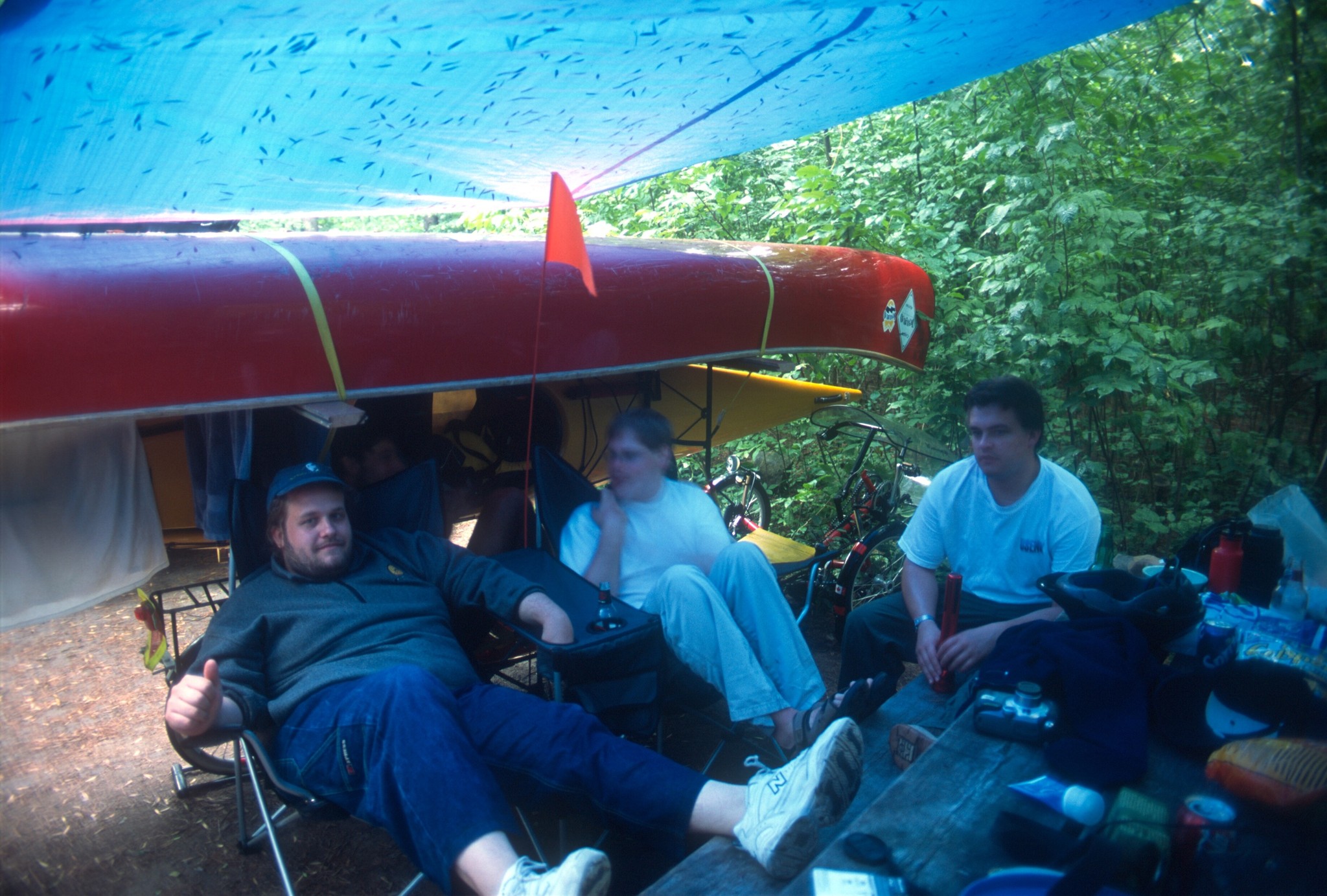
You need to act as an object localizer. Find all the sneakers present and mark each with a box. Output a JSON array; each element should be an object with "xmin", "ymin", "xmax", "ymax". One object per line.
[
  {"xmin": 735, "ymin": 716, "xmax": 866, "ymax": 879},
  {"xmin": 501, "ymin": 847, "xmax": 612, "ymax": 896}
]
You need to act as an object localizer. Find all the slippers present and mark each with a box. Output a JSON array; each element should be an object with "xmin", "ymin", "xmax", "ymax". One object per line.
[{"xmin": 786, "ymin": 672, "xmax": 894, "ymax": 756}]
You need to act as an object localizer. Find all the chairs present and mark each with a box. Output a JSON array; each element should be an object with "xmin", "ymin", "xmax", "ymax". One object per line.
[
  {"xmin": 167, "ymin": 457, "xmax": 552, "ymax": 896},
  {"xmin": 528, "ymin": 449, "xmax": 840, "ymax": 775}
]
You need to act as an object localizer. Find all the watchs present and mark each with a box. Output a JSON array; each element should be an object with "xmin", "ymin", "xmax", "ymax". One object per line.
[{"xmin": 912, "ymin": 614, "xmax": 937, "ymax": 635}]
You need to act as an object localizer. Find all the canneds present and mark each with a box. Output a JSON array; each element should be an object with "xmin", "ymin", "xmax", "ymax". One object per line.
[{"xmin": 1165, "ymin": 794, "xmax": 1237, "ymax": 895}]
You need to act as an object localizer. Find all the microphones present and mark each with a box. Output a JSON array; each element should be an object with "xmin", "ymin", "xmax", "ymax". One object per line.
[{"xmin": 932, "ymin": 573, "xmax": 963, "ymax": 694}]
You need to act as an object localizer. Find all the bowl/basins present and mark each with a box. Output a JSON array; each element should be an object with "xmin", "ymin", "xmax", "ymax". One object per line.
[{"xmin": 1141, "ymin": 565, "xmax": 1208, "ymax": 592}]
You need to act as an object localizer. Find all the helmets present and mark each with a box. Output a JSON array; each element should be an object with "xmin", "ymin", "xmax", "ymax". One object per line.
[{"xmin": 1035, "ymin": 556, "xmax": 1206, "ymax": 640}]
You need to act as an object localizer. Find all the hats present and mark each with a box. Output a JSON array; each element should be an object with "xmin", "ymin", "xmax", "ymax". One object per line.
[{"xmin": 266, "ymin": 459, "xmax": 347, "ymax": 514}]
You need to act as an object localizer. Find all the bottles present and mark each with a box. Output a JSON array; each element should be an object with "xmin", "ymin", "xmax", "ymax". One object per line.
[
  {"xmin": 594, "ymin": 579, "xmax": 619, "ymax": 632},
  {"xmin": 1267, "ymin": 556, "xmax": 1309, "ymax": 622},
  {"xmin": 1205, "ymin": 521, "xmax": 1245, "ymax": 593}
]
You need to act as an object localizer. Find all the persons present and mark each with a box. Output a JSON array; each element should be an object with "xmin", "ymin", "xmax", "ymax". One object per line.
[
  {"xmin": 831, "ymin": 378, "xmax": 1100, "ymax": 694},
  {"xmin": 559, "ymin": 406, "xmax": 892, "ymax": 760},
  {"xmin": 163, "ymin": 433, "xmax": 865, "ymax": 896}
]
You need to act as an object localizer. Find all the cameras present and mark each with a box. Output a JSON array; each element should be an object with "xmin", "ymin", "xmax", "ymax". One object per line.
[{"xmin": 976, "ymin": 683, "xmax": 1059, "ymax": 744}]
